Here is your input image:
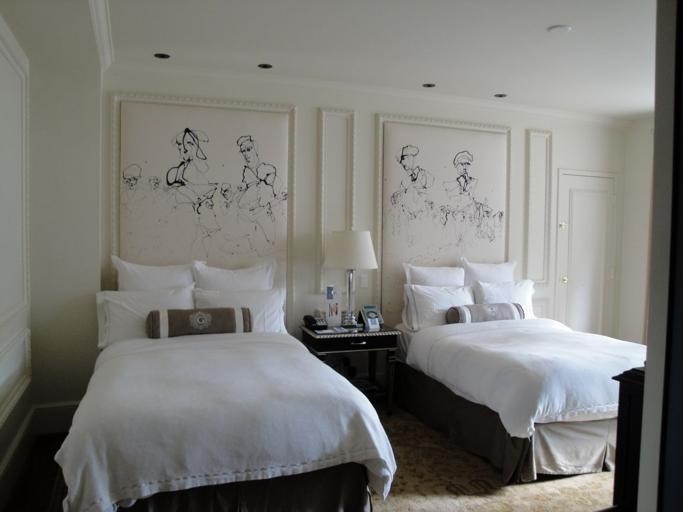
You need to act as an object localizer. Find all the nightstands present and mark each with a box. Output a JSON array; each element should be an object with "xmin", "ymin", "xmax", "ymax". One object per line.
[{"xmin": 299, "ymin": 325, "xmax": 403, "ymax": 417}]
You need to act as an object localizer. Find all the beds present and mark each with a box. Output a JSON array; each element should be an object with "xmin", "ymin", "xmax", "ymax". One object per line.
[
  {"xmin": 397, "ymin": 321, "xmax": 647, "ymax": 483},
  {"xmin": 53, "ymin": 332, "xmax": 399, "ymax": 511}
]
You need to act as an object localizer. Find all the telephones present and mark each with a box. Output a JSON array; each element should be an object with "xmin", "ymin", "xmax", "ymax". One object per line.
[{"xmin": 303, "ymin": 315, "xmax": 328, "ymax": 330}]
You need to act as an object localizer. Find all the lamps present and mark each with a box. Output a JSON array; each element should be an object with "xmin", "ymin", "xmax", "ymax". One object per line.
[{"xmin": 323, "ymin": 229, "xmax": 378, "ymax": 332}]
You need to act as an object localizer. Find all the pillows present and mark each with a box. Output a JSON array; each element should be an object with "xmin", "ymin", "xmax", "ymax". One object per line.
[
  {"xmin": 90, "ymin": 255, "xmax": 287, "ymax": 339},
  {"xmin": 401, "ymin": 262, "xmax": 537, "ymax": 332}
]
